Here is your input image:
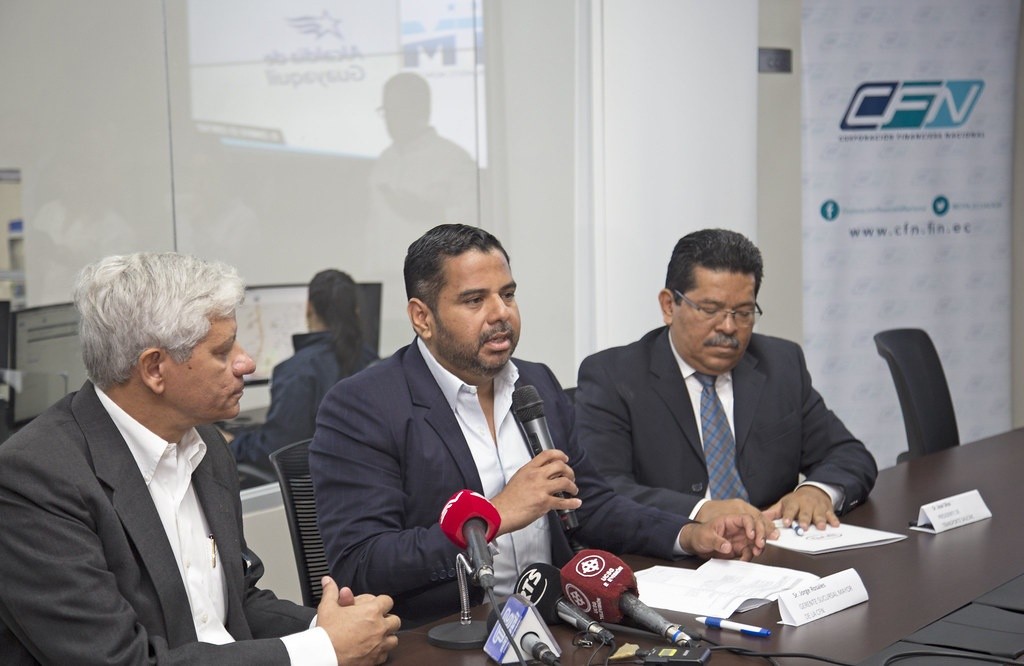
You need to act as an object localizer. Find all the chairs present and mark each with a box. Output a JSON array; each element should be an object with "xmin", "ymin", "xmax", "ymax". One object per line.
[
  {"xmin": 266, "ymin": 437, "xmax": 332, "ymax": 607},
  {"xmin": 874, "ymin": 327, "xmax": 960, "ymax": 465}
]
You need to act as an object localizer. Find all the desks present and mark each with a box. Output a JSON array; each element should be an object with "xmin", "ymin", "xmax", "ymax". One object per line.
[{"xmin": 379, "ymin": 426, "xmax": 1024, "ymax": 666}]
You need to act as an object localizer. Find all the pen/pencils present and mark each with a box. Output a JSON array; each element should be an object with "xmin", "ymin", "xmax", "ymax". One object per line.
[
  {"xmin": 792, "ymin": 520, "xmax": 804, "ymax": 537},
  {"xmin": 694, "ymin": 617, "xmax": 773, "ymax": 638}
]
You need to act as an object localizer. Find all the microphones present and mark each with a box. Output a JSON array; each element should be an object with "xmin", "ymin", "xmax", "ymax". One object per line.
[
  {"xmin": 560, "ymin": 549, "xmax": 701, "ymax": 649},
  {"xmin": 440, "ymin": 489, "xmax": 502, "ymax": 590},
  {"xmin": 514, "ymin": 563, "xmax": 614, "ymax": 645},
  {"xmin": 483, "ymin": 594, "xmax": 562, "ymax": 666},
  {"xmin": 512, "ymin": 386, "xmax": 579, "ymax": 531}
]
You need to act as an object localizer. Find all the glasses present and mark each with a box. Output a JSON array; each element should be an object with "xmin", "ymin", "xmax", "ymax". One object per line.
[{"xmin": 671, "ymin": 287, "xmax": 762, "ymax": 328}]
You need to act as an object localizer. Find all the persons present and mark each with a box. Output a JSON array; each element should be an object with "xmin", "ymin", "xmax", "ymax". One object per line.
[
  {"xmin": 0, "ymin": 252, "xmax": 401, "ymax": 666},
  {"xmin": 309, "ymin": 223, "xmax": 766, "ymax": 633},
  {"xmin": 576, "ymin": 228, "xmax": 879, "ymax": 541},
  {"xmin": 214, "ymin": 268, "xmax": 381, "ymax": 486}
]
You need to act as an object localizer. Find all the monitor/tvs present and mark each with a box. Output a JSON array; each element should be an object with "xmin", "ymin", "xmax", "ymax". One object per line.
[
  {"xmin": 9, "ymin": 302, "xmax": 92, "ymax": 430},
  {"xmin": 232, "ymin": 284, "xmax": 311, "ymax": 386}
]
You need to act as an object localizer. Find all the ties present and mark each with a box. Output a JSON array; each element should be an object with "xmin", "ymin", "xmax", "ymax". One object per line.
[{"xmin": 692, "ymin": 370, "xmax": 749, "ymax": 503}]
